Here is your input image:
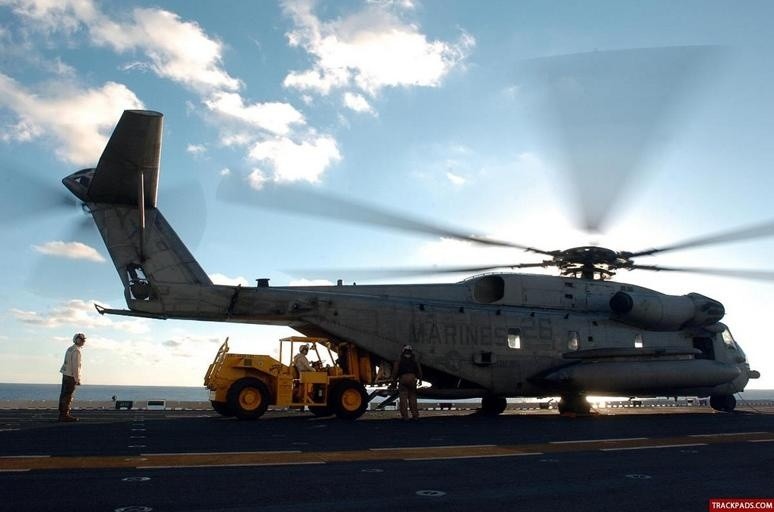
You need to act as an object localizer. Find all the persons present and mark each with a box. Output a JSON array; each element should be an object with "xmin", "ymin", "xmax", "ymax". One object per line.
[
  {"xmin": 389, "ymin": 344, "xmax": 423, "ymax": 421},
  {"xmin": 56, "ymin": 332, "xmax": 85, "ymax": 424},
  {"xmin": 292, "ymin": 342, "xmax": 317, "ymax": 373}
]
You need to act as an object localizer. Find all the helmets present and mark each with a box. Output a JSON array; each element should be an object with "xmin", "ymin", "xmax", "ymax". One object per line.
[
  {"xmin": 300, "ymin": 345, "xmax": 309, "ymax": 354},
  {"xmin": 401, "ymin": 344, "xmax": 413, "ymax": 357},
  {"xmin": 72, "ymin": 333, "xmax": 86, "ymax": 346}
]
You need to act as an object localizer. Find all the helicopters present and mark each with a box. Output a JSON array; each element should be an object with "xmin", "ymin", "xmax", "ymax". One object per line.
[{"xmin": 57, "ymin": 106, "xmax": 772, "ymax": 420}]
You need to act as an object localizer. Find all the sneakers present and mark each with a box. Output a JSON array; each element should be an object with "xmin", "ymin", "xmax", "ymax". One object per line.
[
  {"xmin": 59, "ymin": 415, "xmax": 73, "ymax": 422},
  {"xmin": 412, "ymin": 418, "xmax": 419, "ymax": 422},
  {"xmin": 398, "ymin": 418, "xmax": 409, "ymax": 424}
]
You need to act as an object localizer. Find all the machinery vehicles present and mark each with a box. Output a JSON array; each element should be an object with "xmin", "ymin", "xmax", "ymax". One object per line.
[{"xmin": 201, "ymin": 334, "xmax": 396, "ymax": 422}]
